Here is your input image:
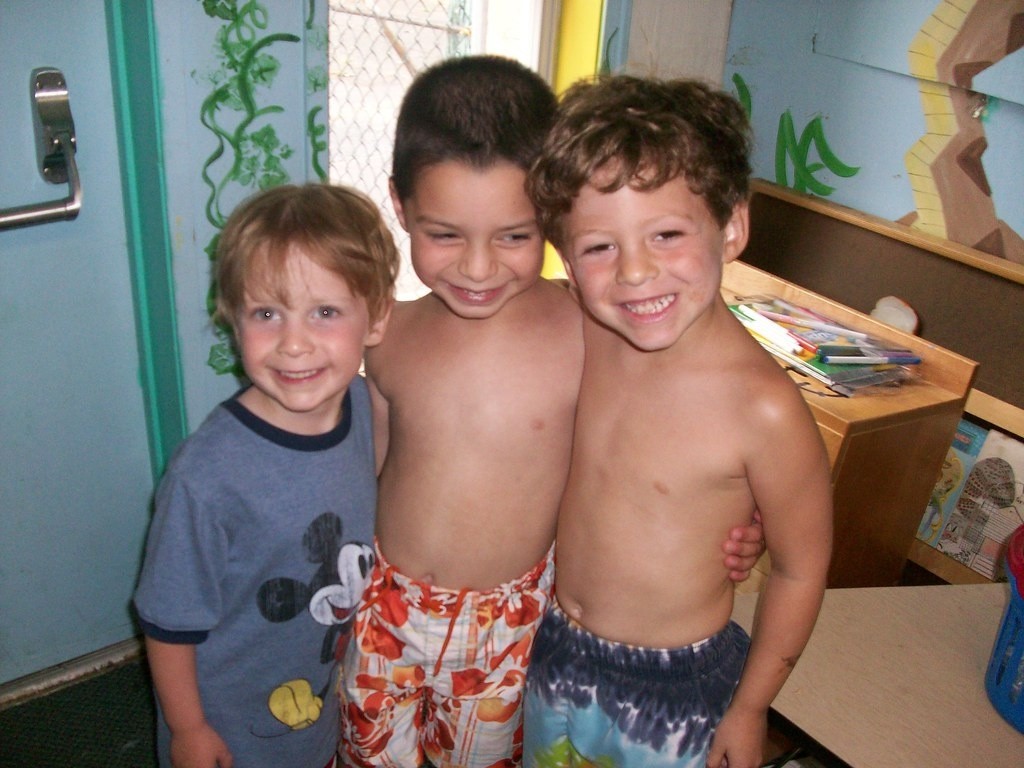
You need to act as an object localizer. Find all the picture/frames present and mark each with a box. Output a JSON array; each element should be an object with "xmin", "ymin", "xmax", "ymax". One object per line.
[{"xmin": 907, "ymin": 385, "xmax": 1024, "ymax": 586}]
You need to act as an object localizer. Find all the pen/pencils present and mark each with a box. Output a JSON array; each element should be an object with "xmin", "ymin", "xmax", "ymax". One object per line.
[
  {"xmin": 758, "ymin": 309, "xmax": 867, "ymax": 342},
  {"xmin": 817, "ymin": 345, "xmax": 921, "ymax": 364}
]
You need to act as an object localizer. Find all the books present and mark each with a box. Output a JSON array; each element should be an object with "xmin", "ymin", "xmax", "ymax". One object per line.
[{"xmin": 727, "ymin": 299, "xmax": 897, "ymax": 388}]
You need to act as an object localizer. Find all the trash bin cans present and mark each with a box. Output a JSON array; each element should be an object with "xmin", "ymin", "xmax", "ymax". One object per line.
[{"xmin": 984, "ymin": 524, "xmax": 1023, "ymax": 735}]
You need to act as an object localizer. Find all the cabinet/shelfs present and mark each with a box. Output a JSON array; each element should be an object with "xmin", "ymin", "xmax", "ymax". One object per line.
[{"xmin": 718, "ymin": 256, "xmax": 980, "ymax": 600}]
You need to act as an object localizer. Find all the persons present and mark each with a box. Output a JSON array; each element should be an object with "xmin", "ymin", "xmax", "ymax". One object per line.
[
  {"xmin": 523, "ymin": 74, "xmax": 833, "ymax": 768},
  {"xmin": 134, "ymin": 184, "xmax": 400, "ymax": 768},
  {"xmin": 335, "ymin": 56, "xmax": 765, "ymax": 768}
]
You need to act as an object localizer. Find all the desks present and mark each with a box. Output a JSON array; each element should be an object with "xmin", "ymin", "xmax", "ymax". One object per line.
[{"xmin": 729, "ymin": 583, "xmax": 1024, "ymax": 768}]
